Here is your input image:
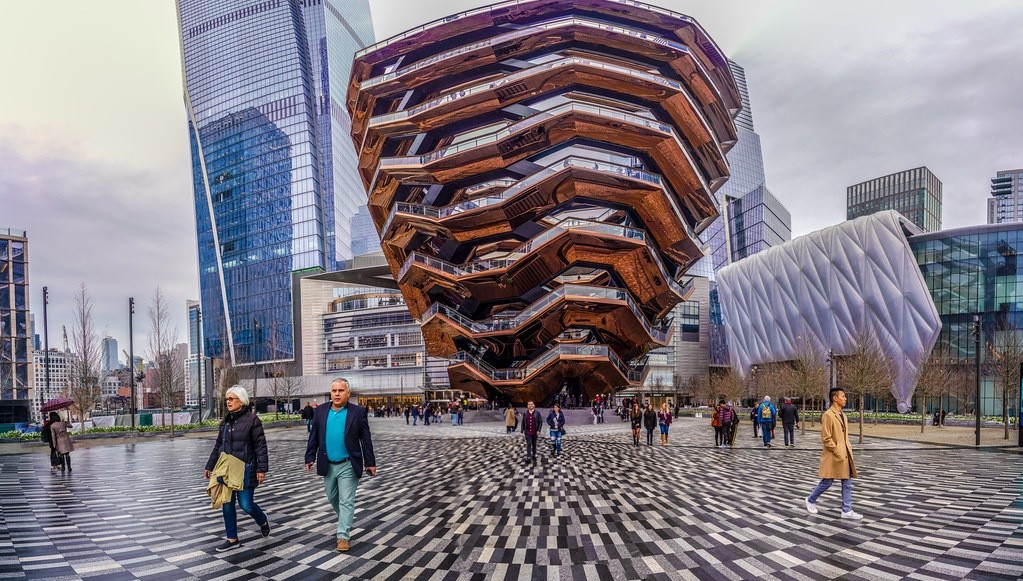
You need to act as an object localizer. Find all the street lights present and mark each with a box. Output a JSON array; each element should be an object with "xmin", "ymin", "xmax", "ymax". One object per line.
[
  {"xmin": 751, "ymin": 366, "xmax": 758, "ymax": 402},
  {"xmin": 972, "ymin": 315, "xmax": 980, "ymax": 445},
  {"xmin": 253, "ymin": 317, "xmax": 257, "ymax": 414},
  {"xmin": 826, "ymin": 348, "xmax": 833, "ymax": 388},
  {"xmin": 43, "ymin": 286, "xmax": 49, "ymax": 402},
  {"xmin": 129, "ymin": 298, "xmax": 135, "ymax": 428}
]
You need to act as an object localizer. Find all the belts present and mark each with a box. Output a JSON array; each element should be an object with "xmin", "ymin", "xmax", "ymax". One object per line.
[{"xmin": 328, "ymin": 457, "xmax": 349, "ymax": 465}]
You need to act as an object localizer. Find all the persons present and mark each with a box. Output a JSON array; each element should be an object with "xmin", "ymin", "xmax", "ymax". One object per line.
[
  {"xmin": 615, "ymin": 398, "xmax": 639, "ymax": 422},
  {"xmin": 375, "ymin": 396, "xmax": 468, "ymax": 426},
  {"xmin": 305, "ymin": 378, "xmax": 377, "ymax": 551},
  {"xmin": 555, "ymin": 393, "xmax": 583, "ymax": 409},
  {"xmin": 591, "ymin": 394, "xmax": 604, "ymax": 424},
  {"xmin": 303, "ymin": 402, "xmax": 315, "ymax": 432},
  {"xmin": 933, "ymin": 409, "xmax": 946, "ymax": 425},
  {"xmin": 44, "ymin": 413, "xmax": 73, "ymax": 472},
  {"xmin": 750, "ymin": 395, "xmax": 800, "ymax": 447},
  {"xmin": 644, "ymin": 404, "xmax": 657, "ymax": 446},
  {"xmin": 503, "ymin": 404, "xmax": 521, "ymax": 433},
  {"xmin": 658, "ymin": 402, "xmax": 672, "ymax": 445},
  {"xmin": 521, "ymin": 401, "xmax": 542, "ymax": 463},
  {"xmin": 711, "ymin": 400, "xmax": 739, "ymax": 446},
  {"xmin": 204, "ymin": 386, "xmax": 270, "ymax": 551},
  {"xmin": 546, "ymin": 404, "xmax": 565, "ymax": 455},
  {"xmin": 674, "ymin": 404, "xmax": 679, "ymax": 419},
  {"xmin": 805, "ymin": 388, "xmax": 863, "ymax": 519},
  {"xmin": 630, "ymin": 404, "xmax": 642, "ymax": 447}
]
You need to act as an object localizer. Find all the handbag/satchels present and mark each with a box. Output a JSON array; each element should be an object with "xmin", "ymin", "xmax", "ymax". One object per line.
[
  {"xmin": 561, "ymin": 428, "xmax": 566, "ymax": 436},
  {"xmin": 659, "ymin": 419, "xmax": 665, "ymax": 425},
  {"xmin": 51, "ymin": 449, "xmax": 62, "ymax": 465},
  {"xmin": 757, "ymin": 427, "xmax": 763, "ymax": 436}
]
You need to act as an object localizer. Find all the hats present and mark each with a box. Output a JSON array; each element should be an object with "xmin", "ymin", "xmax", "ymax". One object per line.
[{"xmin": 784, "ymin": 396, "xmax": 791, "ymax": 402}]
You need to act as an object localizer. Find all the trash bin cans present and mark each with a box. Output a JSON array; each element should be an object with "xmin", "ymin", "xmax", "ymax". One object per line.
[{"xmin": 140, "ymin": 413, "xmax": 152, "ymax": 425}]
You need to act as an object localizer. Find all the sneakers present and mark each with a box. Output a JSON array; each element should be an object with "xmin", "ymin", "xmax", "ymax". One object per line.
[
  {"xmin": 841, "ymin": 510, "xmax": 862, "ymax": 519},
  {"xmin": 805, "ymin": 497, "xmax": 818, "ymax": 514},
  {"xmin": 216, "ymin": 539, "xmax": 240, "ymax": 551},
  {"xmin": 260, "ymin": 513, "xmax": 270, "ymax": 538},
  {"xmin": 337, "ymin": 539, "xmax": 349, "ymax": 551}
]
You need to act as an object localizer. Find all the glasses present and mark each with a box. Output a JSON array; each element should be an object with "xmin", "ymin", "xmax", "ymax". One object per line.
[{"xmin": 224, "ymin": 397, "xmax": 239, "ymax": 402}]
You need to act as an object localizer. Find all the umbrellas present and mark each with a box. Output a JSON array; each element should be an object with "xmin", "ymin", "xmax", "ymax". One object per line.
[{"xmin": 40, "ymin": 398, "xmax": 73, "ymax": 412}]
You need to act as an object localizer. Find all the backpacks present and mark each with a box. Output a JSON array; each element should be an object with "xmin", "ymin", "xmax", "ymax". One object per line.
[
  {"xmin": 40, "ymin": 424, "xmax": 50, "ymax": 441},
  {"xmin": 761, "ymin": 403, "xmax": 772, "ymax": 418}
]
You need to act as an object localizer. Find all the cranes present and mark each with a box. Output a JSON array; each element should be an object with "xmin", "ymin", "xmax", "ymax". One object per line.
[
  {"xmin": 122, "ymin": 349, "xmax": 145, "ymax": 410},
  {"xmin": 62, "ymin": 325, "xmax": 68, "ymax": 353}
]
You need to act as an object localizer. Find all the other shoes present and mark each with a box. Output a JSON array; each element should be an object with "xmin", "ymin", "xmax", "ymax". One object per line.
[
  {"xmin": 533, "ymin": 457, "xmax": 537, "ymax": 462},
  {"xmin": 526, "ymin": 459, "xmax": 530, "ymax": 463},
  {"xmin": 62, "ymin": 466, "xmax": 65, "ymax": 471},
  {"xmin": 68, "ymin": 467, "xmax": 71, "ymax": 471}
]
